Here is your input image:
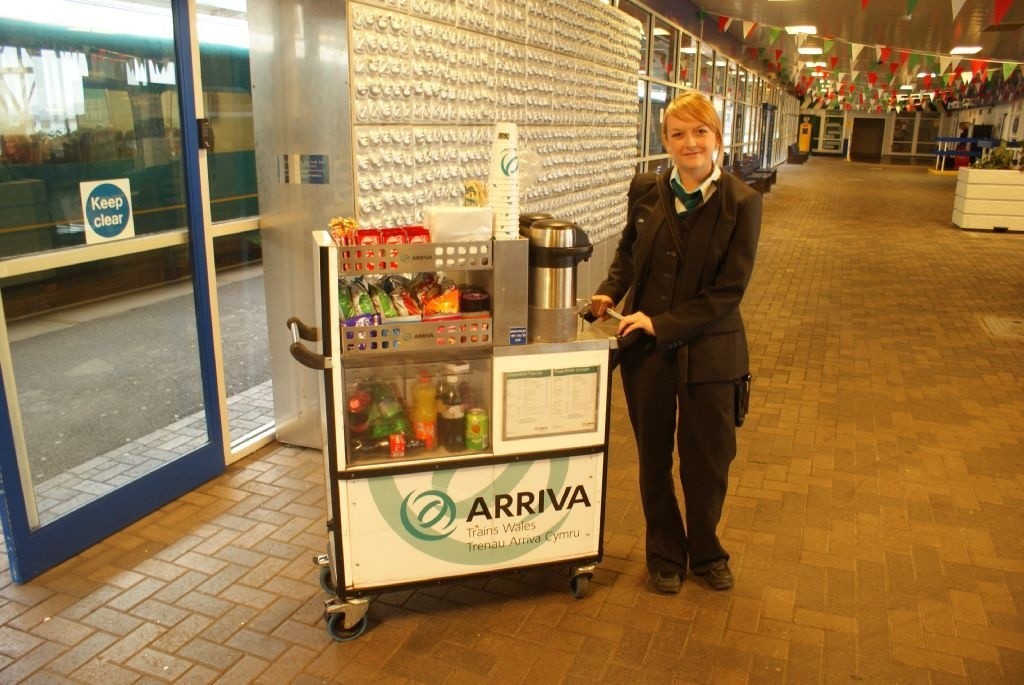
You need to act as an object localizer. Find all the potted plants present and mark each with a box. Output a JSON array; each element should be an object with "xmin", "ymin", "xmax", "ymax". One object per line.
[{"xmin": 951, "ymin": 145, "xmax": 1024, "ymax": 230}]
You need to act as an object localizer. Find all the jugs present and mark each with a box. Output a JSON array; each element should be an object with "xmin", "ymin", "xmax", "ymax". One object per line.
[
  {"xmin": 518, "ymin": 212, "xmax": 554, "ymax": 239},
  {"xmin": 529, "ymin": 219, "xmax": 593, "ymax": 345}
]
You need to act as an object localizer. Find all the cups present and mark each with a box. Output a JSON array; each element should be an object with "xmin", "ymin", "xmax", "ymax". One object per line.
[{"xmin": 489, "ymin": 121, "xmax": 520, "ymax": 240}]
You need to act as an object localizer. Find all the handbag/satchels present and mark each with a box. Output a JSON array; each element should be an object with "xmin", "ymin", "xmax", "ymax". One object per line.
[{"xmin": 736, "ymin": 367, "xmax": 750, "ymax": 427}]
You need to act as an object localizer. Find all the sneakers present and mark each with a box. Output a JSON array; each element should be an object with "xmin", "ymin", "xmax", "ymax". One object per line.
[
  {"xmin": 652, "ymin": 570, "xmax": 683, "ymax": 594},
  {"xmin": 705, "ymin": 564, "xmax": 733, "ymax": 588}
]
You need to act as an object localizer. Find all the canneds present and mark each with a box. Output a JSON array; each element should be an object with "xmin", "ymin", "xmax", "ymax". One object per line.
[
  {"xmin": 460, "ymin": 292, "xmax": 489, "ymax": 341},
  {"xmin": 466, "ymin": 407, "xmax": 489, "ymax": 450}
]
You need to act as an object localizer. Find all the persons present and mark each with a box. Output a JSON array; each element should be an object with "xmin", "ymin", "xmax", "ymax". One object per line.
[{"xmin": 587, "ymin": 92, "xmax": 763, "ymax": 591}]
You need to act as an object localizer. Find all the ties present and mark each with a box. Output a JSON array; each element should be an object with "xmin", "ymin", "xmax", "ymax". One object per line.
[{"xmin": 670, "ymin": 180, "xmax": 703, "ymax": 219}]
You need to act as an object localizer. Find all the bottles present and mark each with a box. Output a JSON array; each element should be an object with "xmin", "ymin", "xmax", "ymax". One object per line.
[
  {"xmin": 345, "ymin": 378, "xmax": 411, "ymax": 453},
  {"xmin": 411, "ymin": 369, "xmax": 428, "ymax": 431},
  {"xmin": 414, "ymin": 375, "xmax": 439, "ymax": 450},
  {"xmin": 437, "ymin": 362, "xmax": 472, "ymax": 453}
]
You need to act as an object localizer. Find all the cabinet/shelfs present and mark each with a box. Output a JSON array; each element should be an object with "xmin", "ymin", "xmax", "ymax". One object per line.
[{"xmin": 330, "ymin": 231, "xmax": 495, "ymax": 355}]
